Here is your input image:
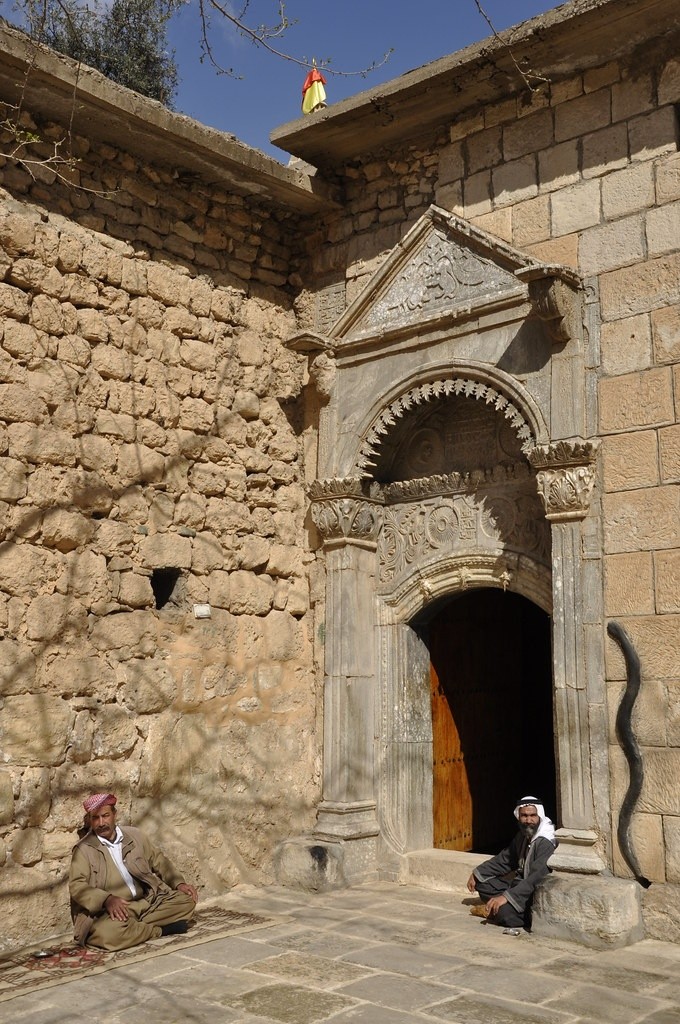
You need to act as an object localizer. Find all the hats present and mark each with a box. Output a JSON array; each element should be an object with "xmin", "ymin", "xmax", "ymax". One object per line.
[{"xmin": 83, "ymin": 793, "xmax": 117, "ymax": 817}]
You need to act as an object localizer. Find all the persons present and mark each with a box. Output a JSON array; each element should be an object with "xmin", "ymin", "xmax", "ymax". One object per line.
[
  {"xmin": 467, "ymin": 796, "xmax": 556, "ymax": 929},
  {"xmin": 69, "ymin": 794, "xmax": 198, "ymax": 953}
]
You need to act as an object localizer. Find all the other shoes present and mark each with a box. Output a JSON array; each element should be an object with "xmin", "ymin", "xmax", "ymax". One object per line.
[
  {"xmin": 470, "ymin": 904, "xmax": 491, "ymax": 919},
  {"xmin": 161, "ymin": 921, "xmax": 187, "ymax": 935}
]
